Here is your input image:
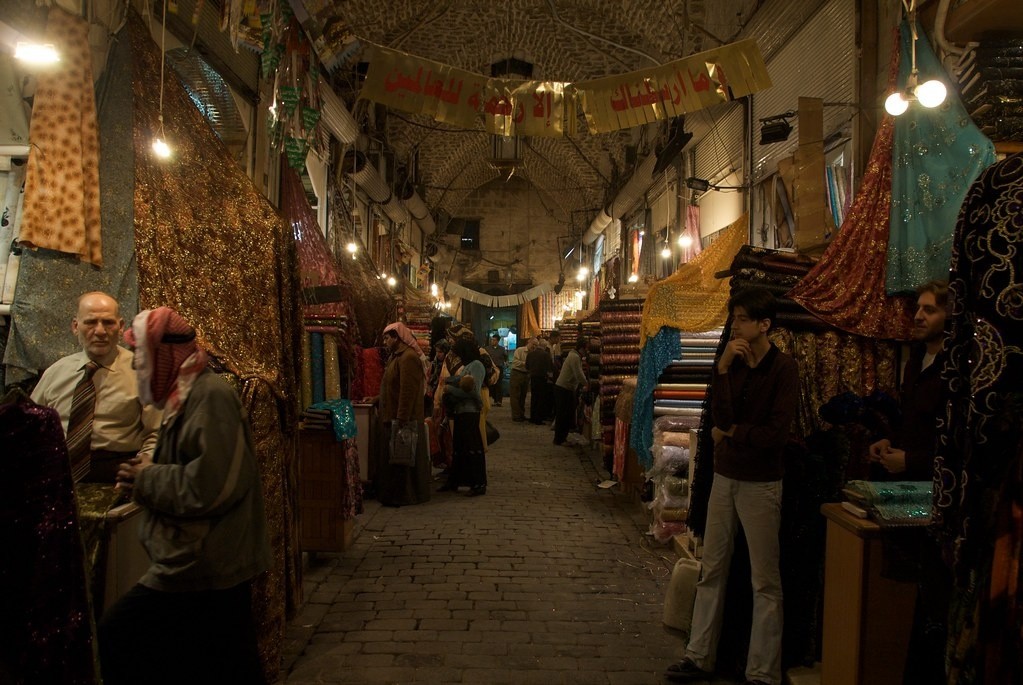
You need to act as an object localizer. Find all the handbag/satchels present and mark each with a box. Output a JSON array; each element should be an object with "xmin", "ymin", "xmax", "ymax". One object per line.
[
  {"xmin": 389, "ymin": 419, "xmax": 417, "ymax": 467},
  {"xmin": 484, "ymin": 421, "xmax": 500, "ymax": 446}
]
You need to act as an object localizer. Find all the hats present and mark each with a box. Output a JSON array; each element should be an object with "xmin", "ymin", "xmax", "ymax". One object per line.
[{"xmin": 124, "ymin": 327, "xmax": 137, "ymax": 348}]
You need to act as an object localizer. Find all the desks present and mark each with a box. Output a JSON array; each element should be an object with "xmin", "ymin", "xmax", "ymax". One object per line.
[{"xmin": 77, "ymin": 483, "xmax": 150, "ymax": 607}]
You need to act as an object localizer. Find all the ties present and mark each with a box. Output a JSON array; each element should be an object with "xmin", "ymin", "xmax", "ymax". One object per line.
[{"xmin": 66, "ymin": 362, "xmax": 100, "ymax": 484}]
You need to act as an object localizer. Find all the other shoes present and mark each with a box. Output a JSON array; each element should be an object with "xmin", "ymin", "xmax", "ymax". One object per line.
[
  {"xmin": 436, "ymin": 482, "xmax": 458, "ymax": 491},
  {"xmin": 497, "ymin": 402, "xmax": 502, "ymax": 406},
  {"xmin": 530, "ymin": 420, "xmax": 544, "ymax": 425},
  {"xmin": 465, "ymin": 485, "xmax": 487, "ymax": 497},
  {"xmin": 524, "ymin": 416, "xmax": 529, "ymax": 420},
  {"xmin": 492, "ymin": 402, "xmax": 497, "ymax": 406},
  {"xmin": 512, "ymin": 418, "xmax": 523, "ymax": 422},
  {"xmin": 662, "ymin": 658, "xmax": 712, "ymax": 677}
]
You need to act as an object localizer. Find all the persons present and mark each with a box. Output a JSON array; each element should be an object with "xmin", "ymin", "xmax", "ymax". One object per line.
[
  {"xmin": 485, "ymin": 334, "xmax": 510, "ymax": 406},
  {"xmin": 553, "ymin": 339, "xmax": 588, "ymax": 448},
  {"xmin": 526, "ymin": 339, "xmax": 553, "ymax": 425},
  {"xmin": 429, "ymin": 325, "xmax": 500, "ymax": 496},
  {"xmin": 362, "ymin": 322, "xmax": 431, "ymax": 505},
  {"xmin": 870, "ymin": 280, "xmax": 948, "ymax": 482},
  {"xmin": 30, "ymin": 291, "xmax": 162, "ymax": 482},
  {"xmin": 664, "ymin": 288, "xmax": 800, "ymax": 685},
  {"xmin": 98, "ymin": 306, "xmax": 270, "ymax": 685},
  {"xmin": 510, "ymin": 337, "xmax": 539, "ymax": 422},
  {"xmin": 547, "ymin": 331, "xmax": 560, "ymax": 354}
]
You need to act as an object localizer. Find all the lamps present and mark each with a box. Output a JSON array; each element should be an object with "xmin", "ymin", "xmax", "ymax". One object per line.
[
  {"xmin": 553, "ymin": 236, "xmax": 574, "ymax": 294},
  {"xmin": 685, "ymin": 177, "xmax": 746, "ymax": 193},
  {"xmin": 482, "ymin": 134, "xmax": 526, "ymax": 182},
  {"xmin": 758, "ymin": 100, "xmax": 870, "ymax": 146},
  {"xmin": 497, "ymin": 321, "xmax": 510, "ymax": 338},
  {"xmin": 501, "ymin": 262, "xmax": 519, "ymax": 288}
]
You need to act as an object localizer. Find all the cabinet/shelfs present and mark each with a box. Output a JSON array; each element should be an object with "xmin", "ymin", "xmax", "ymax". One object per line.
[
  {"xmin": 814, "ymin": 500, "xmax": 944, "ymax": 685},
  {"xmin": 299, "ymin": 419, "xmax": 357, "ymax": 569},
  {"xmin": 353, "ymin": 402, "xmax": 391, "ymax": 485}
]
